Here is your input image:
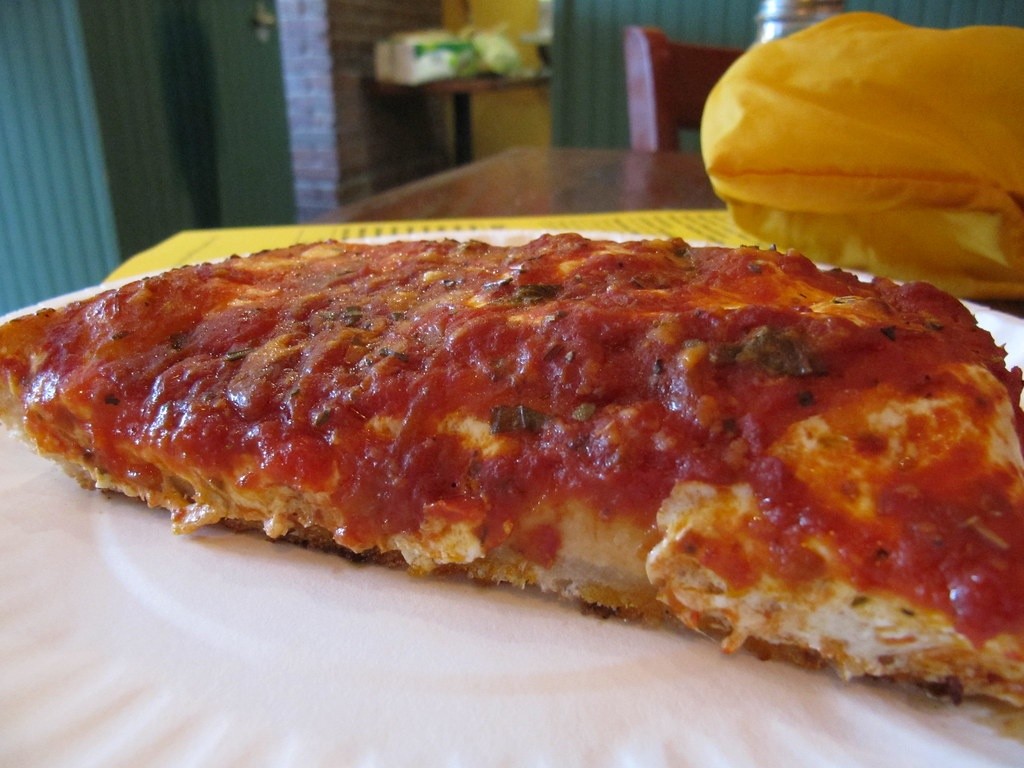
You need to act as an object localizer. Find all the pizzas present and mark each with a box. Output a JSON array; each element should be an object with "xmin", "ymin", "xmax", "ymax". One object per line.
[{"xmin": 1, "ymin": 230, "xmax": 1024, "ymax": 737}]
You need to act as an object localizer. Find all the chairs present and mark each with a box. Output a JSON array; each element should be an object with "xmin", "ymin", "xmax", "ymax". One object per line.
[{"xmin": 623, "ymin": 25, "xmax": 745, "ymax": 157}]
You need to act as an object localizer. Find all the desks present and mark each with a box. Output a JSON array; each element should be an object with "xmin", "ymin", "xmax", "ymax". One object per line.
[{"xmin": 432, "ymin": 74, "xmax": 549, "ymax": 167}]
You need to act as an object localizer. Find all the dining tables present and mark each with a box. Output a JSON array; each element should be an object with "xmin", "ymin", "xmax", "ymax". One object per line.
[{"xmin": 0, "ymin": 145, "xmax": 1024, "ymax": 768}]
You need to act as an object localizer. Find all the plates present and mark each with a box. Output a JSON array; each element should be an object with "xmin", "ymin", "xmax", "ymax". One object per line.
[{"xmin": 0, "ymin": 229, "xmax": 1024, "ymax": 768}]
